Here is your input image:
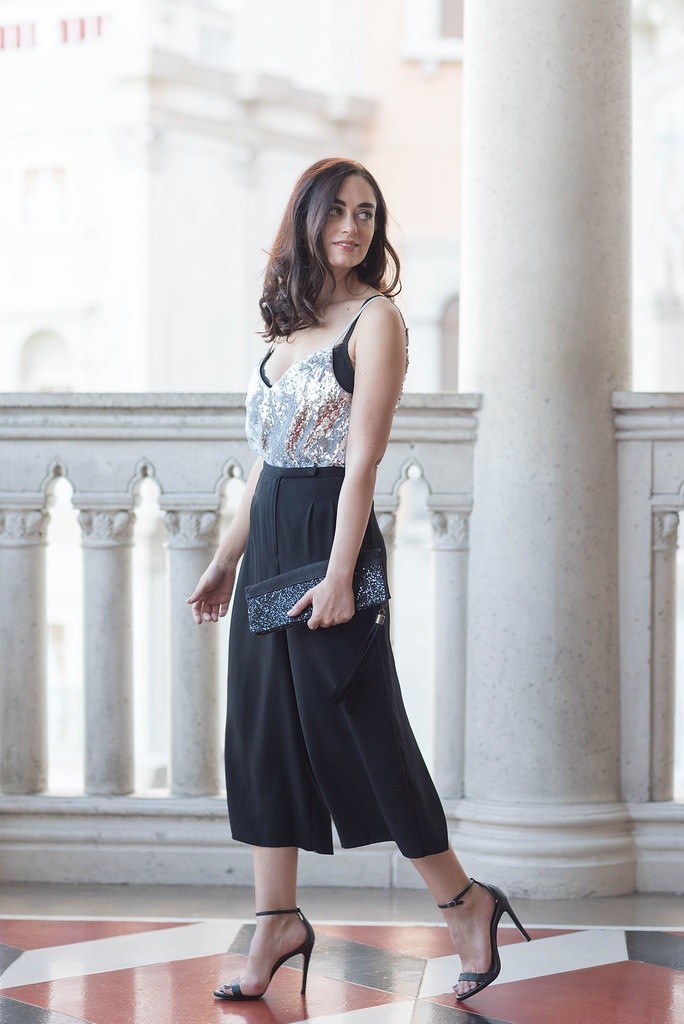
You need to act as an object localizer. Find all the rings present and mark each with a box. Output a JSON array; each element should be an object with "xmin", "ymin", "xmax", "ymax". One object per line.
[{"xmin": 306, "ymin": 621, "xmax": 318, "ymax": 630}]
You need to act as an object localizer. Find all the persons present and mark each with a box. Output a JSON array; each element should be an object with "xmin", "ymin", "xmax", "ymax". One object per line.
[{"xmin": 187, "ymin": 159, "xmax": 529, "ymax": 1000}]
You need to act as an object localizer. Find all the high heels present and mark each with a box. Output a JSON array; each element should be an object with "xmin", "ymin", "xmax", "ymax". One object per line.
[
  {"xmin": 213, "ymin": 905, "xmax": 315, "ymax": 1000},
  {"xmin": 436, "ymin": 877, "xmax": 531, "ymax": 1001}
]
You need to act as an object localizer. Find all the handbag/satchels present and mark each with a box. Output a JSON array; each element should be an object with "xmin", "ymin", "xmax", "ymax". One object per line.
[{"xmin": 244, "ymin": 546, "xmax": 396, "ymax": 710}]
